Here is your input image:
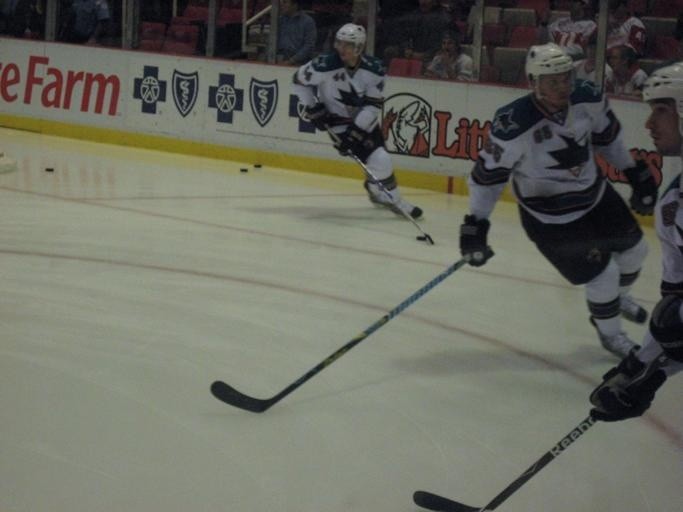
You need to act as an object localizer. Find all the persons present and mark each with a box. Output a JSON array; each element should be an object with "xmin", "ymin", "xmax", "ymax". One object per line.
[
  {"xmin": 590, "ymin": 61, "xmax": 683, "ymax": 422},
  {"xmin": 293, "ymin": 23, "xmax": 423, "ymax": 218},
  {"xmin": 0, "ymin": 0, "xmax": 683, "ymax": 100},
  {"xmin": 459, "ymin": 44, "xmax": 647, "ymax": 357}
]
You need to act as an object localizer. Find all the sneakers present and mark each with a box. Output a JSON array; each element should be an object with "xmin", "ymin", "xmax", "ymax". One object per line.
[
  {"xmin": 621, "ymin": 296, "xmax": 647, "ymax": 324},
  {"xmin": 600, "ymin": 331, "xmax": 641, "ymax": 358}
]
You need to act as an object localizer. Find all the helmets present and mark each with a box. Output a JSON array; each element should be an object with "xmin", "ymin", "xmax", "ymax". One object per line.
[
  {"xmin": 643, "ymin": 62, "xmax": 682, "ymax": 120},
  {"xmin": 334, "ymin": 23, "xmax": 366, "ymax": 57},
  {"xmin": 525, "ymin": 42, "xmax": 577, "ymax": 97}
]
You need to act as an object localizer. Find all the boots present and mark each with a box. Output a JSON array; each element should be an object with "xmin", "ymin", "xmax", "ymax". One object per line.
[{"xmin": 364, "ymin": 172, "xmax": 422, "ymax": 219}]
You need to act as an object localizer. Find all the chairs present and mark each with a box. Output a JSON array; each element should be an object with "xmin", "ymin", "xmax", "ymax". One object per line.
[{"xmin": 1, "ymin": 0, "xmax": 682, "ymax": 102}]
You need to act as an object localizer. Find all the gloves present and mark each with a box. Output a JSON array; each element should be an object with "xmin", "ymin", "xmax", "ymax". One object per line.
[
  {"xmin": 588, "ymin": 353, "xmax": 667, "ymax": 422},
  {"xmin": 459, "ymin": 213, "xmax": 491, "ymax": 268},
  {"xmin": 306, "ymin": 102, "xmax": 333, "ymax": 131},
  {"xmin": 338, "ymin": 123, "xmax": 367, "ymax": 157},
  {"xmin": 621, "ymin": 158, "xmax": 657, "ymax": 216}
]
